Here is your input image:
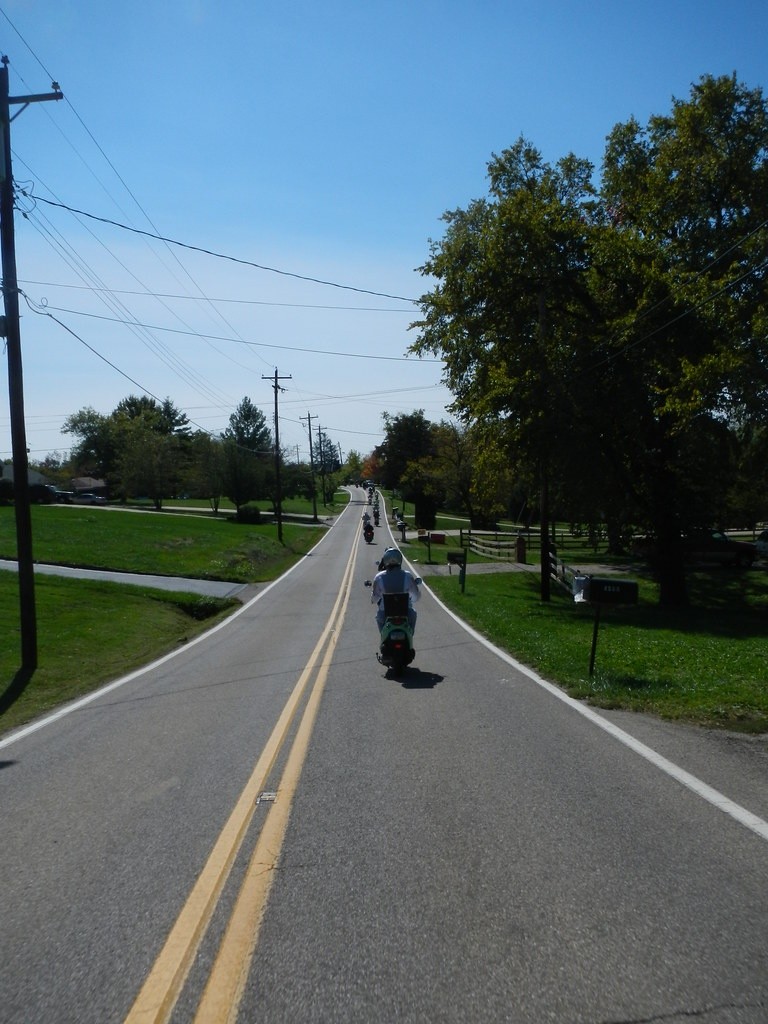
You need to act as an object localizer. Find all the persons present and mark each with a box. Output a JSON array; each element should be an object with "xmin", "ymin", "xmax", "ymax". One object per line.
[
  {"xmin": 370, "ymin": 546, "xmax": 421, "ymax": 634},
  {"xmin": 362, "ymin": 484, "xmax": 380, "ymax": 539}
]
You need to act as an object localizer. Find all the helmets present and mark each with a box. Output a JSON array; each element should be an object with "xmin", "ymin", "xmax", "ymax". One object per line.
[
  {"xmin": 382, "ymin": 549, "xmax": 402, "ymax": 567},
  {"xmin": 366, "ymin": 521, "xmax": 370, "ymax": 525},
  {"xmin": 364, "ymin": 512, "xmax": 368, "ymax": 515}
]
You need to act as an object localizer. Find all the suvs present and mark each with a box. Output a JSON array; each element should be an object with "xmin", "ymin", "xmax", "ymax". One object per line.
[{"xmin": 37, "ymin": 484, "xmax": 75, "ymax": 503}]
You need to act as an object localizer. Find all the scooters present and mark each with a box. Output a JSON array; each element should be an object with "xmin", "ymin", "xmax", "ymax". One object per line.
[
  {"xmin": 367, "ymin": 491, "xmax": 380, "ymax": 527},
  {"xmin": 361, "ymin": 515, "xmax": 372, "ymax": 528},
  {"xmin": 363, "ymin": 529, "xmax": 374, "ymax": 544},
  {"xmin": 364, "ymin": 576, "xmax": 423, "ymax": 677}
]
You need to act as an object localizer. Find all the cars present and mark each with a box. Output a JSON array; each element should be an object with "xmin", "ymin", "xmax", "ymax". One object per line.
[
  {"xmin": 364, "ymin": 480, "xmax": 374, "ymax": 487},
  {"xmin": 68, "ymin": 494, "xmax": 108, "ymax": 506}
]
